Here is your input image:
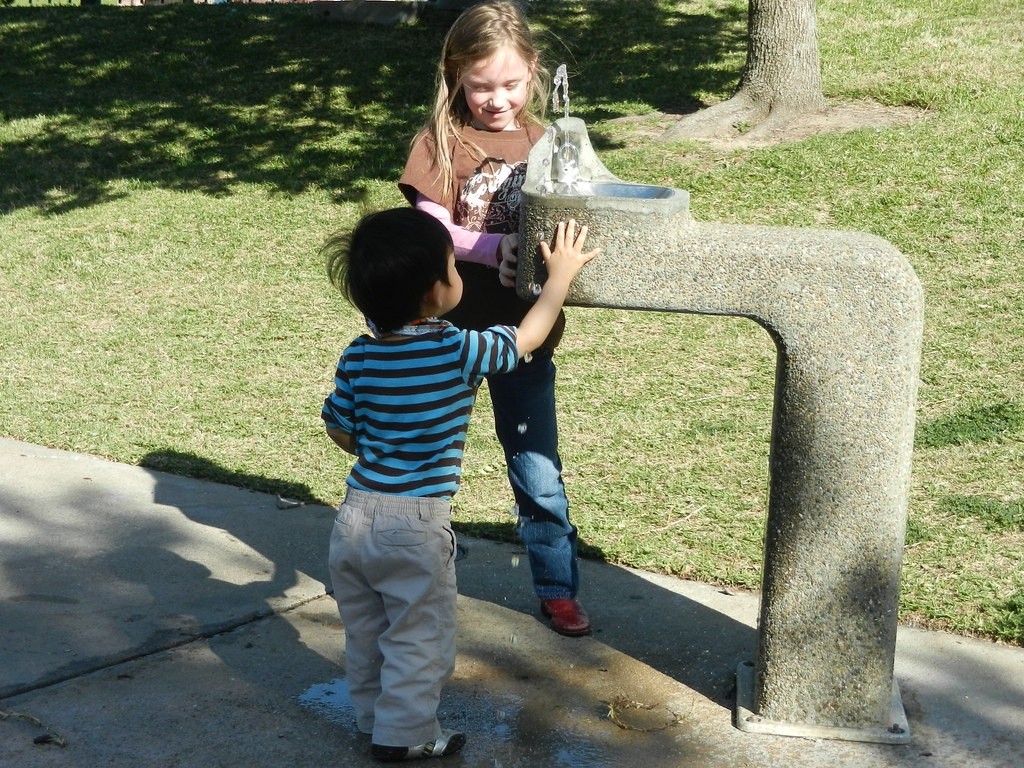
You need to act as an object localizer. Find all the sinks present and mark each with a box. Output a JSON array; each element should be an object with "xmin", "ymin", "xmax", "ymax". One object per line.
[{"xmin": 535, "ymin": 174, "xmax": 689, "ymax": 222}]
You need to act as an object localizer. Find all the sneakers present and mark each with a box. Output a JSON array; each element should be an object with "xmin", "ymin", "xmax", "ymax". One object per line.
[{"xmin": 370, "ymin": 728, "xmax": 466, "ymax": 762}]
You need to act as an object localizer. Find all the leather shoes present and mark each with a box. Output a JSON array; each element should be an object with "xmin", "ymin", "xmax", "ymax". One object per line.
[{"xmin": 544, "ymin": 598, "xmax": 592, "ymax": 636}]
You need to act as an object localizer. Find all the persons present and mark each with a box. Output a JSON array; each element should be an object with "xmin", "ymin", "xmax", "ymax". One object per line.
[
  {"xmin": 393, "ymin": 0, "xmax": 595, "ymax": 642},
  {"xmin": 317, "ymin": 209, "xmax": 606, "ymax": 759}
]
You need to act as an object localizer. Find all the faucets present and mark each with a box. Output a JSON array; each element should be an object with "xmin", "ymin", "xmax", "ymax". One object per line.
[{"xmin": 553, "ymin": 140, "xmax": 591, "ymax": 183}]
